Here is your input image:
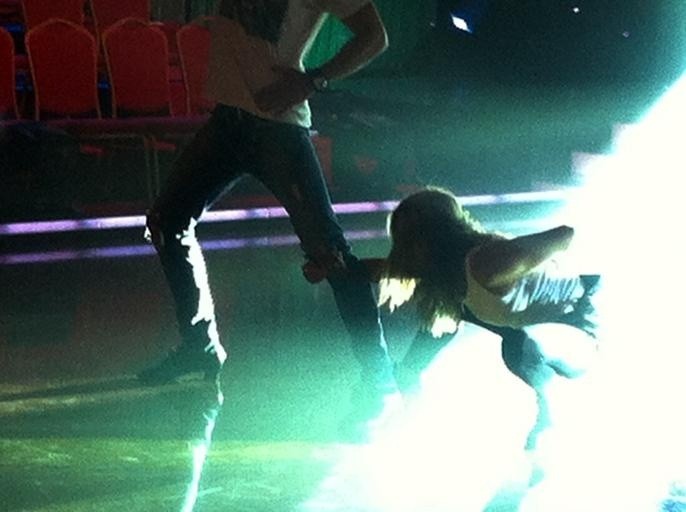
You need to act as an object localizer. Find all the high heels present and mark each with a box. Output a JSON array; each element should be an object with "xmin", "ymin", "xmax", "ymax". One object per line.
[{"xmin": 135, "ymin": 344, "xmax": 227, "ymax": 389}]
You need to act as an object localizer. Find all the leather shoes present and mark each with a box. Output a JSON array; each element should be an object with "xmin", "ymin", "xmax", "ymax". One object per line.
[{"xmin": 358, "ymin": 361, "xmax": 404, "ymax": 409}]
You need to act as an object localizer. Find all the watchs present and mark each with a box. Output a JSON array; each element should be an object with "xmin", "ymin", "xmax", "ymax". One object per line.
[{"xmin": 309, "ymin": 67, "xmax": 328, "ymax": 93}]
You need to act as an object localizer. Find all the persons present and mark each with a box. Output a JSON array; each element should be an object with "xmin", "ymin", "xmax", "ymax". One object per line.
[
  {"xmin": 135, "ymin": 0, "xmax": 392, "ymax": 398},
  {"xmin": 300, "ymin": 188, "xmax": 606, "ymax": 332}
]
